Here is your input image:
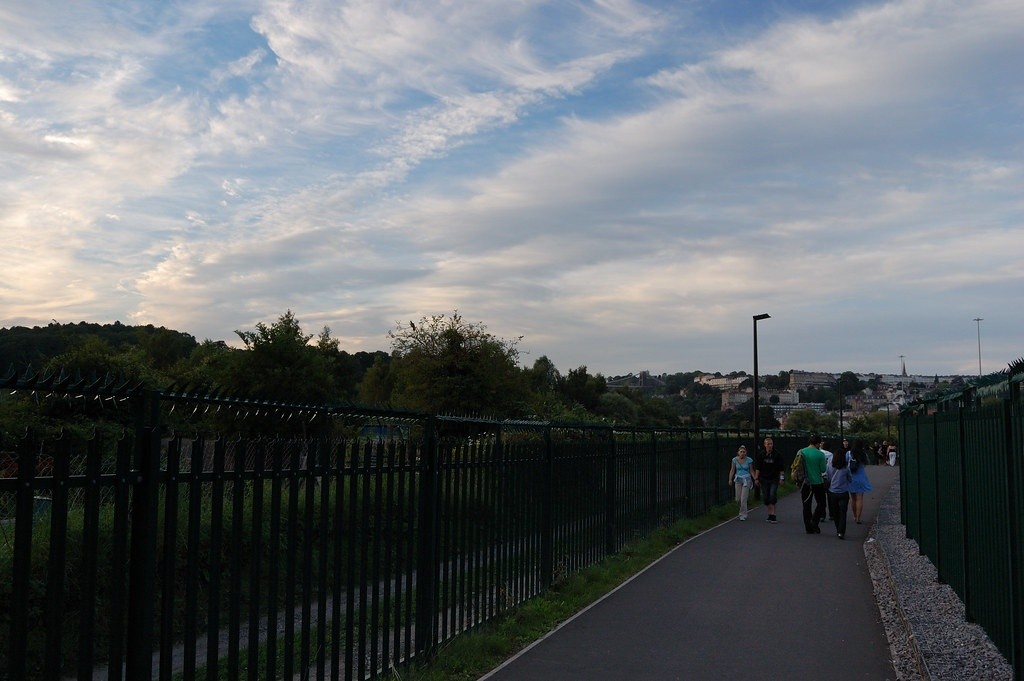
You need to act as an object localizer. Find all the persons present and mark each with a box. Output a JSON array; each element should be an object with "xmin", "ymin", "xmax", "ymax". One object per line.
[
  {"xmin": 797, "ymin": 434, "xmax": 874, "ymax": 539},
  {"xmin": 729, "ymin": 438, "xmax": 784, "ymax": 522},
  {"xmin": 870, "ymin": 441, "xmax": 896, "ymax": 467}
]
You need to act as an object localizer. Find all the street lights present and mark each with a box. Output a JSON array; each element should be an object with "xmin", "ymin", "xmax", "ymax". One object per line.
[
  {"xmin": 898, "ymin": 356, "xmax": 905, "ymax": 398},
  {"xmin": 888, "ymin": 403, "xmax": 891, "ymax": 442},
  {"xmin": 753, "ymin": 313, "xmax": 772, "ymax": 501},
  {"xmin": 839, "ymin": 380, "xmax": 847, "ymax": 449},
  {"xmin": 972, "ymin": 318, "xmax": 984, "ymax": 379}
]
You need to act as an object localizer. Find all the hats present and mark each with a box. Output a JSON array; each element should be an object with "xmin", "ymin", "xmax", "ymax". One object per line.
[{"xmin": 843, "ymin": 438, "xmax": 848, "ymax": 441}]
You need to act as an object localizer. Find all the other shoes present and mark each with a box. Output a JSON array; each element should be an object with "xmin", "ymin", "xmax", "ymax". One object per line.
[
  {"xmin": 829, "ymin": 517, "xmax": 834, "ymax": 520},
  {"xmin": 806, "ymin": 530, "xmax": 811, "ymax": 533},
  {"xmin": 820, "ymin": 518, "xmax": 824, "ymax": 522},
  {"xmin": 838, "ymin": 533, "xmax": 844, "ymax": 538},
  {"xmin": 771, "ymin": 514, "xmax": 777, "ymax": 523},
  {"xmin": 740, "ymin": 516, "xmax": 745, "ymax": 520},
  {"xmin": 766, "ymin": 515, "xmax": 771, "ymax": 521},
  {"xmin": 856, "ymin": 520, "xmax": 862, "ymax": 524},
  {"xmin": 810, "ymin": 520, "xmax": 821, "ymax": 534}
]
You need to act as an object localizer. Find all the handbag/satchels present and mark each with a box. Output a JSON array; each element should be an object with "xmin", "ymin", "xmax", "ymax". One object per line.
[
  {"xmin": 791, "ymin": 449, "xmax": 806, "ymax": 483},
  {"xmin": 848, "ymin": 450, "xmax": 859, "ymax": 472}
]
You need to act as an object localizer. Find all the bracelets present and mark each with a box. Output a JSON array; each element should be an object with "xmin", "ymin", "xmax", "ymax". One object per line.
[
  {"xmin": 780, "ymin": 476, "xmax": 784, "ymax": 479},
  {"xmin": 755, "ymin": 478, "xmax": 758, "ymax": 480}
]
